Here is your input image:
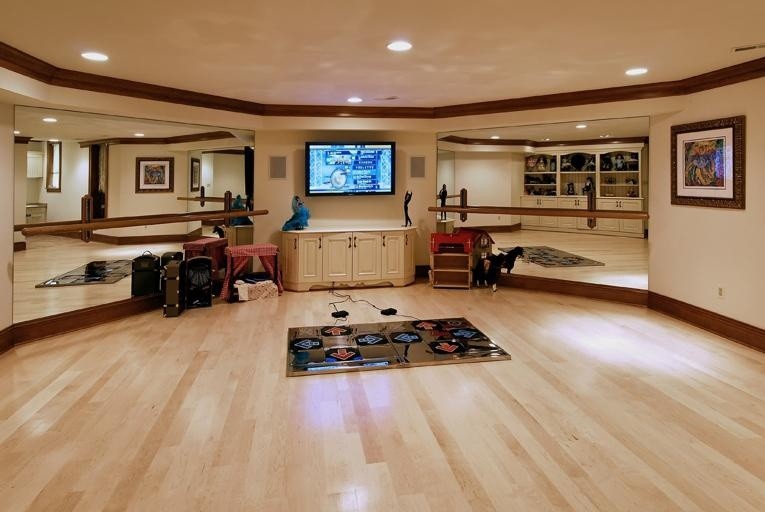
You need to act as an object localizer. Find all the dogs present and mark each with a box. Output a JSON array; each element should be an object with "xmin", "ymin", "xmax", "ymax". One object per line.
[
  {"xmin": 476, "ymin": 253, "xmax": 506, "ymax": 292},
  {"xmin": 501, "ymin": 246, "xmax": 524, "ymax": 274}
]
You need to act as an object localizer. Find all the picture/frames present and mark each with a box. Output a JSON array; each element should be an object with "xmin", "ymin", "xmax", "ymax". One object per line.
[
  {"xmin": 670, "ymin": 114, "xmax": 745, "ymax": 210},
  {"xmin": 134, "ymin": 156, "xmax": 175, "ymax": 193},
  {"xmin": 191, "ymin": 158, "xmax": 200, "ymax": 191}
]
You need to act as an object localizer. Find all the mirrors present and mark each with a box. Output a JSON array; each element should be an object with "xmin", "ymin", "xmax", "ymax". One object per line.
[
  {"xmin": 435, "ymin": 117, "xmax": 650, "ymax": 292},
  {"xmin": 13, "ymin": 102, "xmax": 254, "ymax": 329}
]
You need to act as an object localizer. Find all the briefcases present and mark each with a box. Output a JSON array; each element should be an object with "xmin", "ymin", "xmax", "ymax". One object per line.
[
  {"xmin": 163, "ymin": 260, "xmax": 184, "ymax": 317},
  {"xmin": 131, "ymin": 251, "xmax": 160, "ymax": 296}
]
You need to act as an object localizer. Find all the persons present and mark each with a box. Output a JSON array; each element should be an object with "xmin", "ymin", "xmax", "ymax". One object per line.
[
  {"xmin": 230, "ymin": 195, "xmax": 254, "ymax": 226},
  {"xmin": 404, "ymin": 190, "xmax": 412, "ymax": 227},
  {"xmin": 282, "ymin": 195, "xmax": 311, "ymax": 231},
  {"xmin": 439, "ymin": 184, "xmax": 447, "ymax": 220}
]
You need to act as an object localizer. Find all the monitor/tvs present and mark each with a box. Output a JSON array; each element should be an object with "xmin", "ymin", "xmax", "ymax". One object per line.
[
  {"xmin": 244, "ymin": 146, "xmax": 254, "ymax": 201},
  {"xmin": 305, "ymin": 141, "xmax": 396, "ymax": 197}
]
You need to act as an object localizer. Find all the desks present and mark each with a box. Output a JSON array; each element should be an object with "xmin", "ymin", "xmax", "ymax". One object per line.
[
  {"xmin": 183, "ymin": 238, "xmax": 228, "ymax": 279},
  {"xmin": 220, "ymin": 245, "xmax": 283, "ymax": 303}
]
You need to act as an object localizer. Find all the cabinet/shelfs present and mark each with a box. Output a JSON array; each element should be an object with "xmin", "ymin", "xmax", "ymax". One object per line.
[
  {"xmin": 201, "ymin": 224, "xmax": 253, "ymax": 278},
  {"xmin": 520, "ymin": 142, "xmax": 644, "ymax": 238},
  {"xmin": 430, "ymin": 252, "xmax": 470, "ymax": 289},
  {"xmin": 437, "ymin": 221, "xmax": 456, "ymax": 235},
  {"xmin": 279, "ymin": 229, "xmax": 415, "ymax": 292}
]
